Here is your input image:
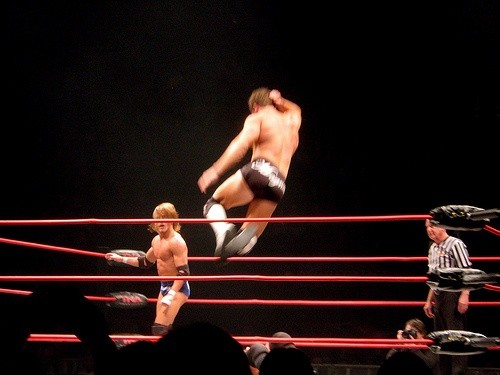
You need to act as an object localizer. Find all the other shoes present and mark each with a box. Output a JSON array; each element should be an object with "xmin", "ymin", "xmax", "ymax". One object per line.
[
  {"xmin": 225, "ymin": 227, "xmax": 256, "ymax": 254},
  {"xmin": 213, "ymin": 223, "xmax": 237, "ymax": 256}
]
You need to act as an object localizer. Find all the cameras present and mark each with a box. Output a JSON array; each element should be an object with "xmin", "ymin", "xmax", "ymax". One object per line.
[{"xmin": 401, "ymin": 329, "xmax": 417, "ymax": 339}]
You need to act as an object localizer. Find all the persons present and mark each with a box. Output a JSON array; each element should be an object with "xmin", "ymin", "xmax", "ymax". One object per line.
[
  {"xmin": 384, "ymin": 316, "xmax": 439, "ymax": 375},
  {"xmin": 0, "ymin": 259, "xmax": 314, "ymax": 375},
  {"xmin": 105, "ymin": 203, "xmax": 191, "ymax": 337},
  {"xmin": 423, "ymin": 216, "xmax": 472, "ymax": 331},
  {"xmin": 197, "ymin": 88, "xmax": 302, "ymax": 258}
]
